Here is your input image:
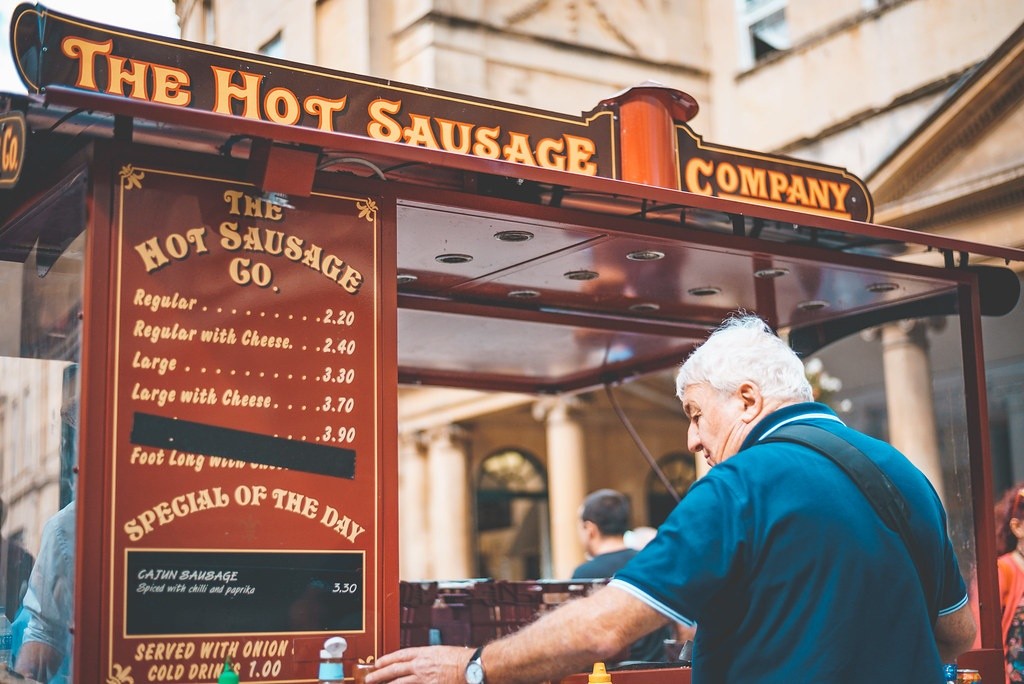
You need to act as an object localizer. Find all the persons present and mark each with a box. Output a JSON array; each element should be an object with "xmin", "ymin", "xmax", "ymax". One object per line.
[
  {"xmin": 365, "ymin": 315, "xmax": 976, "ymax": 684},
  {"xmin": 969, "ymin": 481, "xmax": 1024, "ymax": 684},
  {"xmin": 0, "ymin": 464, "xmax": 78, "ymax": 684}
]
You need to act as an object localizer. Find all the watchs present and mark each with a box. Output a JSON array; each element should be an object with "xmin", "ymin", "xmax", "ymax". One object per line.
[{"xmin": 465, "ymin": 646, "xmax": 485, "ymax": 684}]
